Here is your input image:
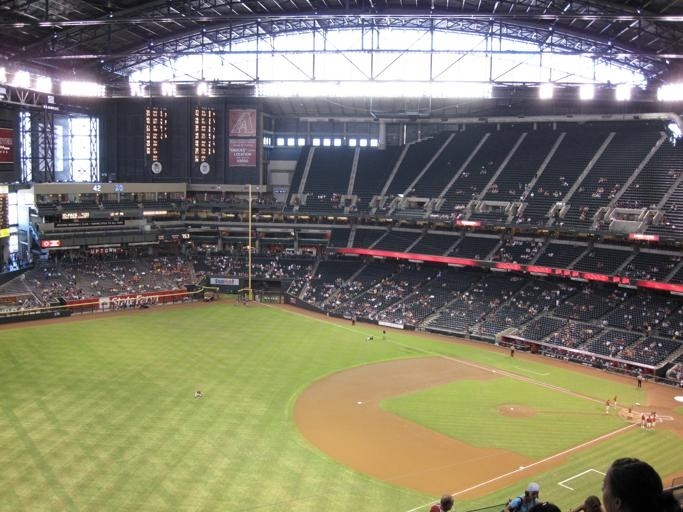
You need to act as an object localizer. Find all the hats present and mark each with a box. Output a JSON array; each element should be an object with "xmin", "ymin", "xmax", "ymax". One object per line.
[{"xmin": 525, "ymin": 482, "xmax": 540, "ymax": 492}]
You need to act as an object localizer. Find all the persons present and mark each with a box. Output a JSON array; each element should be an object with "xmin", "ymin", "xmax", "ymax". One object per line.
[
  {"xmin": 627, "ymin": 407, "xmax": 632, "ymax": 418},
  {"xmin": 637, "ymin": 374, "xmax": 642, "ymax": 388},
  {"xmin": 254, "ymin": 161, "xmax": 682, "ymax": 384},
  {"xmin": 605, "ymin": 395, "xmax": 617, "ymax": 414},
  {"xmin": 430, "ymin": 494, "xmax": 454, "ymax": 512},
  {"xmin": 2, "ymin": 248, "xmax": 249, "ymax": 312},
  {"xmin": 503, "ymin": 458, "xmax": 683, "ymax": 512},
  {"xmin": 641, "ymin": 412, "xmax": 657, "ymax": 431}
]
